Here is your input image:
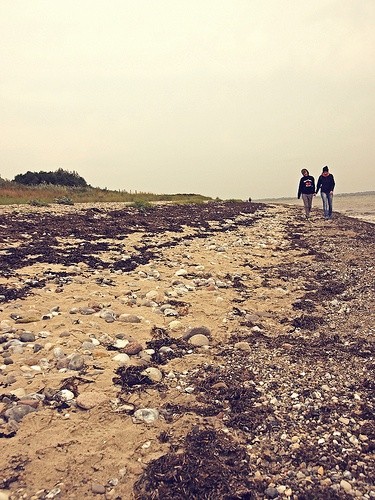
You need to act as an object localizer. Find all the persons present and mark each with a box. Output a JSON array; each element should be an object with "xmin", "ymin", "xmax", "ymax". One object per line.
[
  {"xmin": 297, "ymin": 169, "xmax": 315, "ymax": 219},
  {"xmin": 313, "ymin": 166, "xmax": 335, "ymax": 219}
]
